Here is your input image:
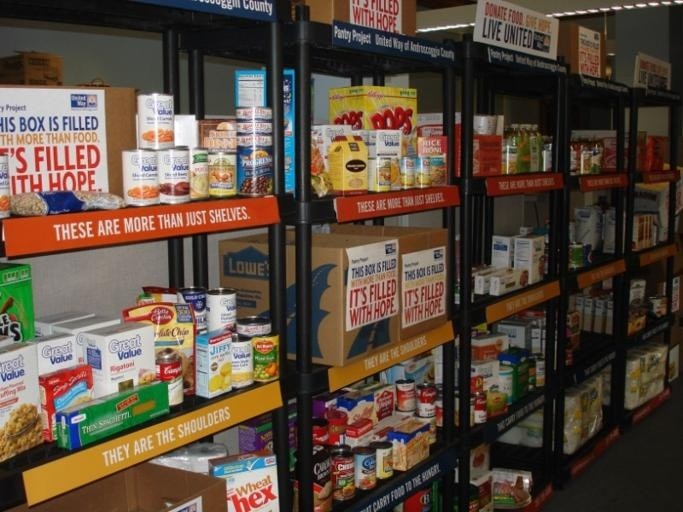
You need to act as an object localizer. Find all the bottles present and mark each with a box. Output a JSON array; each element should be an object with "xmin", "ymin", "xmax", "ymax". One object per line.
[{"xmin": 502, "ymin": 123, "xmax": 604, "ymax": 177}]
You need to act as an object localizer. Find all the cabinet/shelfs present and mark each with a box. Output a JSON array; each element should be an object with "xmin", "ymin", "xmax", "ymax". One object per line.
[{"xmin": 0, "ymin": 0, "xmax": 683, "ymax": 512}]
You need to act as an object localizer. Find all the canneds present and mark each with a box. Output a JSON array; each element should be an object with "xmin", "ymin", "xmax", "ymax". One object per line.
[
  {"xmin": 400, "ymin": 155, "xmax": 445, "ymax": 190},
  {"xmin": 498, "ymin": 366, "xmax": 517, "ymax": 406},
  {"xmin": 155, "ymin": 348, "xmax": 184, "ymax": 408},
  {"xmin": 455, "ymin": 392, "xmax": 487, "ymax": 427},
  {"xmin": 569, "ymin": 241, "xmax": 592, "ymax": 270},
  {"xmin": 473, "ymin": 114, "xmax": 498, "ymax": 135},
  {"xmin": 312, "ymin": 416, "xmax": 394, "ymax": 503},
  {"xmin": 528, "ymin": 353, "xmax": 545, "ymax": 392},
  {"xmin": 121, "ymin": 91, "xmax": 273, "ymax": 207},
  {"xmin": 395, "ymin": 378, "xmax": 438, "ymax": 445},
  {"xmin": 542, "ymin": 135, "xmax": 604, "ymax": 175},
  {"xmin": 178, "ymin": 287, "xmax": 279, "ymax": 389}
]
